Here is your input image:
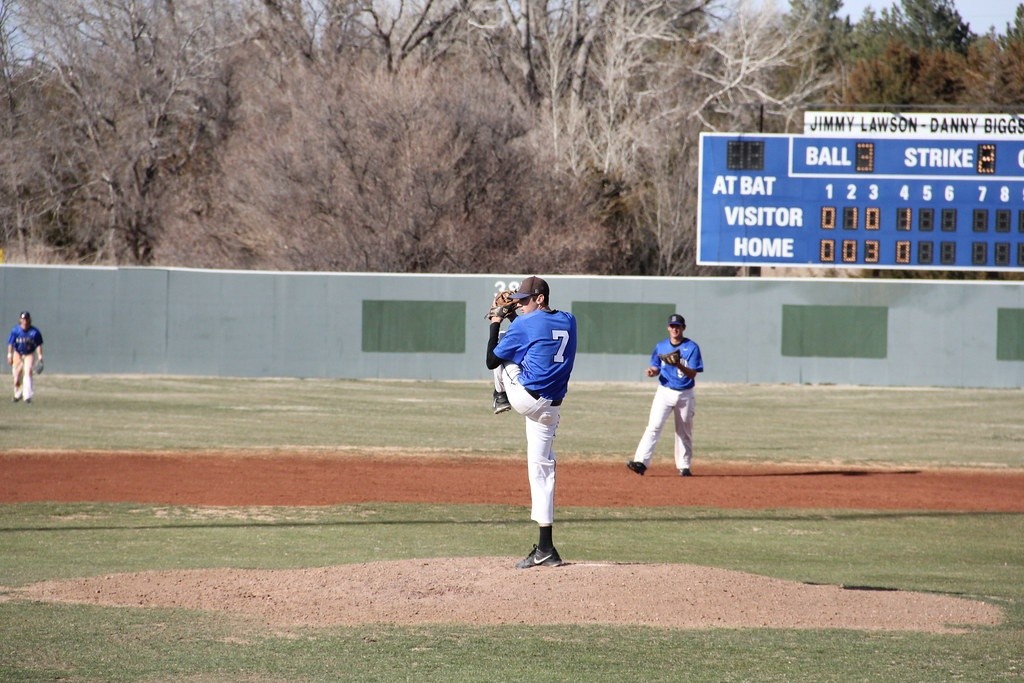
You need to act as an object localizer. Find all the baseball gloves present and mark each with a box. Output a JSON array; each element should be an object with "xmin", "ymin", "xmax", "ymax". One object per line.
[
  {"xmin": 657, "ymin": 348, "xmax": 681, "ymax": 365},
  {"xmin": 31, "ymin": 359, "xmax": 44, "ymax": 374},
  {"xmin": 487, "ymin": 291, "xmax": 519, "ymax": 319}
]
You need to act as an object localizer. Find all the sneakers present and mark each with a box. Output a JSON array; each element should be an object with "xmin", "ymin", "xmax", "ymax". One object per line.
[
  {"xmin": 492, "ymin": 389, "xmax": 511, "ymax": 414},
  {"xmin": 515, "ymin": 544, "xmax": 562, "ymax": 570}
]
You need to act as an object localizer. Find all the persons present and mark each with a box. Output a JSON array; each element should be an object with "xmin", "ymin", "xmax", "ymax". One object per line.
[
  {"xmin": 627, "ymin": 314, "xmax": 704, "ymax": 476},
  {"xmin": 486, "ymin": 276, "xmax": 577, "ymax": 568},
  {"xmin": 7, "ymin": 311, "xmax": 43, "ymax": 403}
]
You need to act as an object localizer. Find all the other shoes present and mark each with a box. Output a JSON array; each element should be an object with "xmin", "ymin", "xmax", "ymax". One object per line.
[
  {"xmin": 25, "ymin": 399, "xmax": 31, "ymax": 403},
  {"xmin": 626, "ymin": 461, "xmax": 647, "ymax": 475},
  {"xmin": 12, "ymin": 396, "xmax": 22, "ymax": 402},
  {"xmin": 682, "ymin": 469, "xmax": 692, "ymax": 476}
]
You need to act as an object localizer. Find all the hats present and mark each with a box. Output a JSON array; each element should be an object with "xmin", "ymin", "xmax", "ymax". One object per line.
[
  {"xmin": 20, "ymin": 311, "xmax": 30, "ymax": 319},
  {"xmin": 668, "ymin": 314, "xmax": 685, "ymax": 325},
  {"xmin": 507, "ymin": 276, "xmax": 549, "ymax": 299}
]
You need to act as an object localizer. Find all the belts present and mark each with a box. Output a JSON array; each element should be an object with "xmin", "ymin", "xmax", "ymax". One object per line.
[{"xmin": 525, "ymin": 387, "xmax": 562, "ymax": 406}]
